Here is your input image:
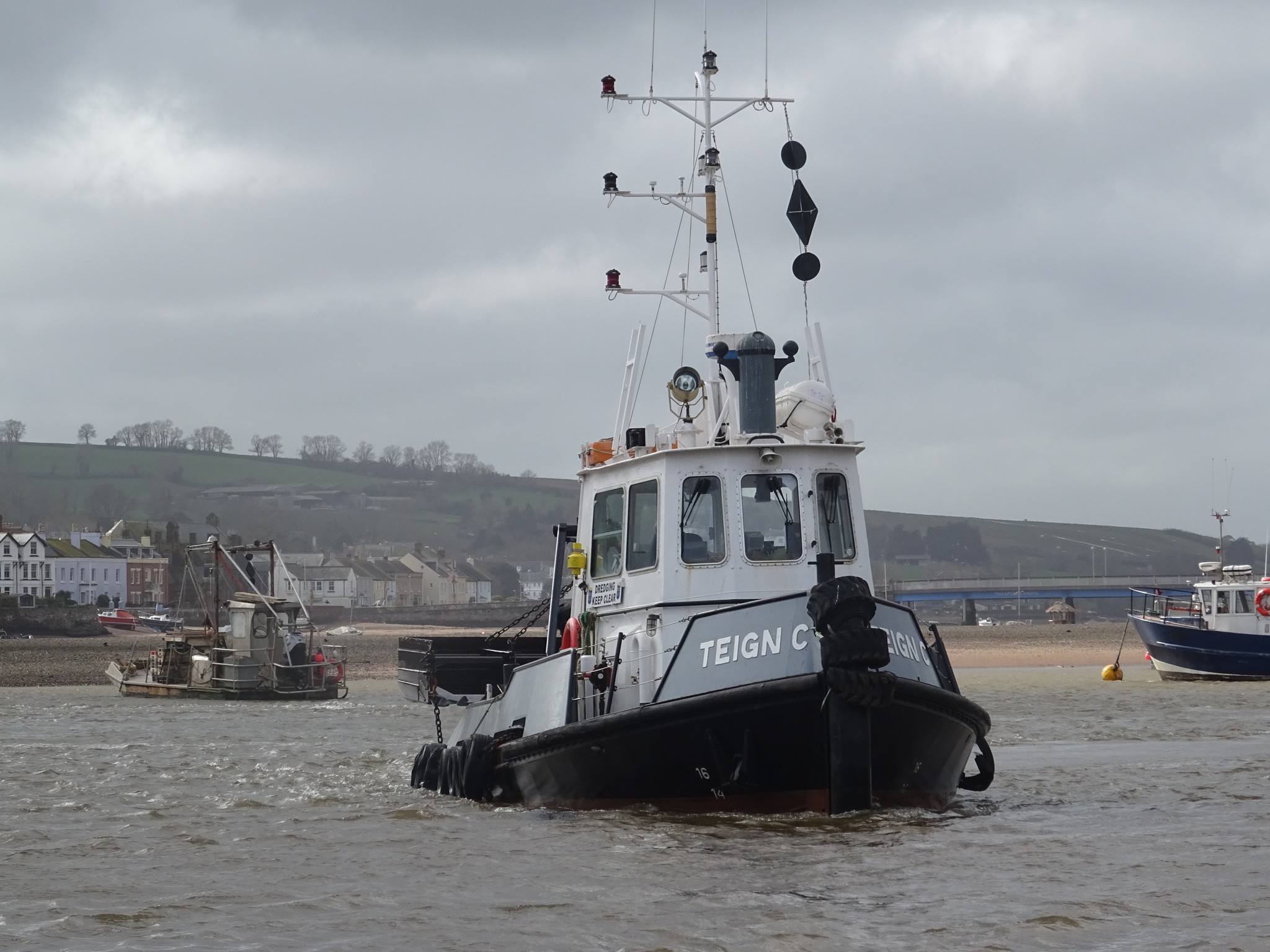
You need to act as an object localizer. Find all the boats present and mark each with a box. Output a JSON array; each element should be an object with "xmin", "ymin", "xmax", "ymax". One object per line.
[
  {"xmin": 393, "ymin": 3, "xmax": 996, "ymax": 820},
  {"xmin": 1129, "ymin": 560, "xmax": 1270, "ymax": 683},
  {"xmin": 115, "ymin": 537, "xmax": 346, "ymax": 701}
]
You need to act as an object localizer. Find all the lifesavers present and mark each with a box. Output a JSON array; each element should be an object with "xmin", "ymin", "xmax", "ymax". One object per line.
[
  {"xmin": 1254, "ymin": 588, "xmax": 1270, "ymax": 617},
  {"xmin": 320, "ymin": 659, "xmax": 343, "ymax": 683},
  {"xmin": 559, "ymin": 616, "xmax": 579, "ymax": 649},
  {"xmin": 410, "ymin": 734, "xmax": 494, "ymax": 802}
]
